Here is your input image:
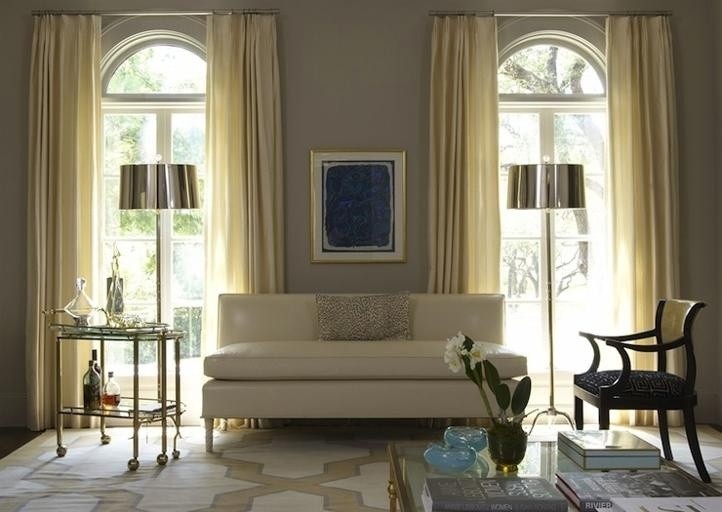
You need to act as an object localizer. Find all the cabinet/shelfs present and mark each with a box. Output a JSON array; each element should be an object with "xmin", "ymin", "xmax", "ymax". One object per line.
[{"xmin": 49, "ymin": 321, "xmax": 188, "ymax": 469}]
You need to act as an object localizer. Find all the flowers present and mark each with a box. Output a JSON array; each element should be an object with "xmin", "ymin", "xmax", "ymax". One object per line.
[{"xmin": 439, "ymin": 331, "xmax": 533, "ymax": 421}]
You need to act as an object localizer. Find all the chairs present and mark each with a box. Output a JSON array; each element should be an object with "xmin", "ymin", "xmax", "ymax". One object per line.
[{"xmin": 571, "ymin": 296, "xmax": 715, "ymax": 486}]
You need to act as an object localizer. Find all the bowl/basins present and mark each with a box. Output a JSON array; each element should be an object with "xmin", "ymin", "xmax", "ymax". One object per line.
[
  {"xmin": 445, "ymin": 424, "xmax": 488, "ymax": 449},
  {"xmin": 472, "ymin": 453, "xmax": 491, "ymax": 478},
  {"xmin": 422, "ymin": 445, "xmax": 478, "ymax": 474}
]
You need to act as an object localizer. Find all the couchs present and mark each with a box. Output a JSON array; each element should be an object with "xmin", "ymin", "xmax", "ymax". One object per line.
[{"xmin": 200, "ymin": 291, "xmax": 527, "ymax": 455}]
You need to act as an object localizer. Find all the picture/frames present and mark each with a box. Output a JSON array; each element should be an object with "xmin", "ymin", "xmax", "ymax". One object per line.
[{"xmin": 308, "ymin": 149, "xmax": 407, "ymax": 264}]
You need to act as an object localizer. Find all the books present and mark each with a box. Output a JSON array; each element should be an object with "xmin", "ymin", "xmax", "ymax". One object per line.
[
  {"xmin": 557, "ymin": 430, "xmax": 661, "ymax": 470},
  {"xmin": 421, "ymin": 477, "xmax": 568, "ymax": 512},
  {"xmin": 555, "ymin": 469, "xmax": 722, "ymax": 512}
]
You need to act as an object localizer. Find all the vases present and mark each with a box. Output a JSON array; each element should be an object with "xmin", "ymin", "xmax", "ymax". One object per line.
[{"xmin": 486, "ymin": 421, "xmax": 528, "ymax": 471}]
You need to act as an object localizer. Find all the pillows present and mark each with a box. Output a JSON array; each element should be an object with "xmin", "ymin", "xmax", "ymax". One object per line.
[{"xmin": 313, "ymin": 287, "xmax": 413, "ymax": 342}]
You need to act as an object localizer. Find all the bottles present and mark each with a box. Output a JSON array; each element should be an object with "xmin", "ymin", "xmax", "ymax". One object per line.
[
  {"xmin": 62, "ymin": 277, "xmax": 98, "ymax": 324},
  {"xmin": 82, "ymin": 349, "xmax": 121, "ymax": 412}
]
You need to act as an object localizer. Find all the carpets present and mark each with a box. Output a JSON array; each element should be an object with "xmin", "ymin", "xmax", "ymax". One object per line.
[{"xmin": 0, "ymin": 422, "xmax": 722, "ymax": 512}]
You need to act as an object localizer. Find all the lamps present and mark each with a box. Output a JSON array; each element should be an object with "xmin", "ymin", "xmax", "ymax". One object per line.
[
  {"xmin": 119, "ymin": 153, "xmax": 203, "ymax": 405},
  {"xmin": 506, "ymin": 156, "xmax": 589, "ymax": 437}
]
